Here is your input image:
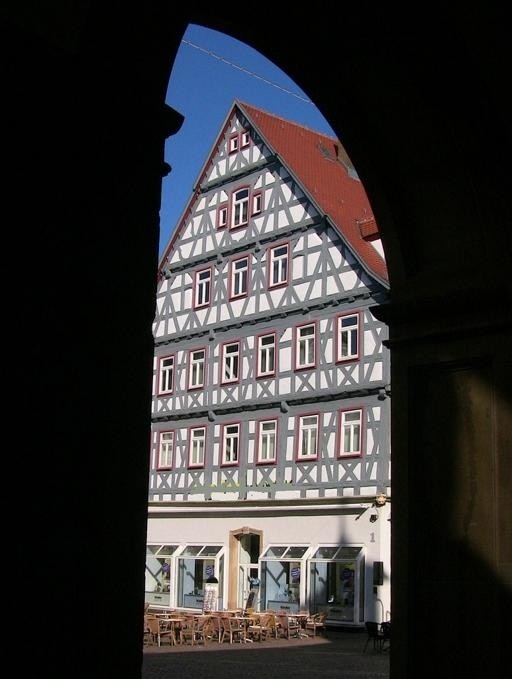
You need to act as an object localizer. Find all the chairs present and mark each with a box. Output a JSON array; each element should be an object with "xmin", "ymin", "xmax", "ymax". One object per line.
[
  {"xmin": 143, "ymin": 601, "xmax": 329, "ymax": 649},
  {"xmin": 361, "ymin": 621, "xmax": 391, "ymax": 654}
]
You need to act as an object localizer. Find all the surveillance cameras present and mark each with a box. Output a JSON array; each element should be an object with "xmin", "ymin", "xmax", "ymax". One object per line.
[{"xmin": 370, "ymin": 508, "xmax": 377, "ymax": 520}]
